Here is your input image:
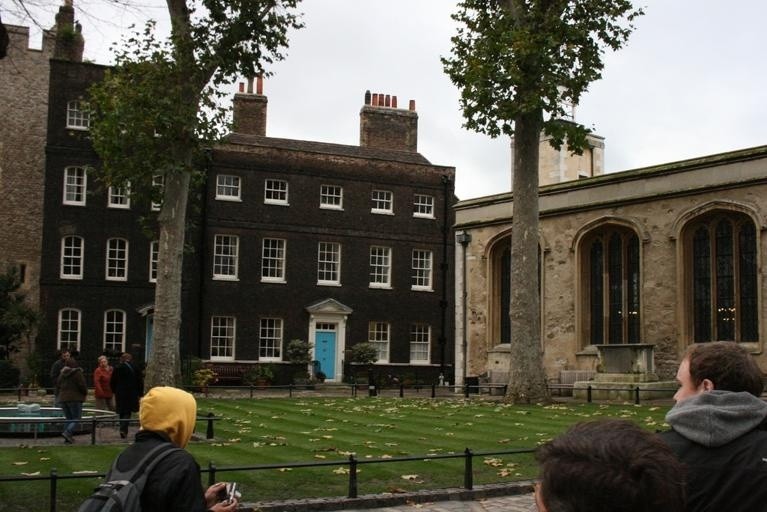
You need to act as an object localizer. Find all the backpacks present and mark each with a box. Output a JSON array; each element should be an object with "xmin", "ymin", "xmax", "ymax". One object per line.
[{"xmin": 80, "ymin": 444, "xmax": 184, "ymax": 512}]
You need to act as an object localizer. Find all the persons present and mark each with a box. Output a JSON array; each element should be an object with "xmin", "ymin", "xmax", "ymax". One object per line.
[
  {"xmin": 654, "ymin": 340, "xmax": 767, "ymax": 512},
  {"xmin": 49, "ymin": 349, "xmax": 71, "ymax": 408},
  {"xmin": 531, "ymin": 416, "xmax": 687, "ymax": 512},
  {"xmin": 72, "ymin": 347, "xmax": 80, "ymax": 359},
  {"xmin": 110, "ymin": 353, "xmax": 142, "ymax": 438},
  {"xmin": 109, "ymin": 386, "xmax": 238, "ymax": 512},
  {"xmin": 92, "ymin": 356, "xmax": 114, "ymax": 416},
  {"xmin": 56, "ymin": 359, "xmax": 88, "ymax": 444}
]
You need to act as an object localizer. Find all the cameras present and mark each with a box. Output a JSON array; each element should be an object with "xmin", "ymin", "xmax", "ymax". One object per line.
[{"xmin": 226, "ymin": 482, "xmax": 242, "ymax": 505}]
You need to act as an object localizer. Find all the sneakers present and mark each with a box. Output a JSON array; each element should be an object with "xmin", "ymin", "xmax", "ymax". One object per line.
[{"xmin": 62, "ymin": 431, "xmax": 74, "ymax": 444}]
